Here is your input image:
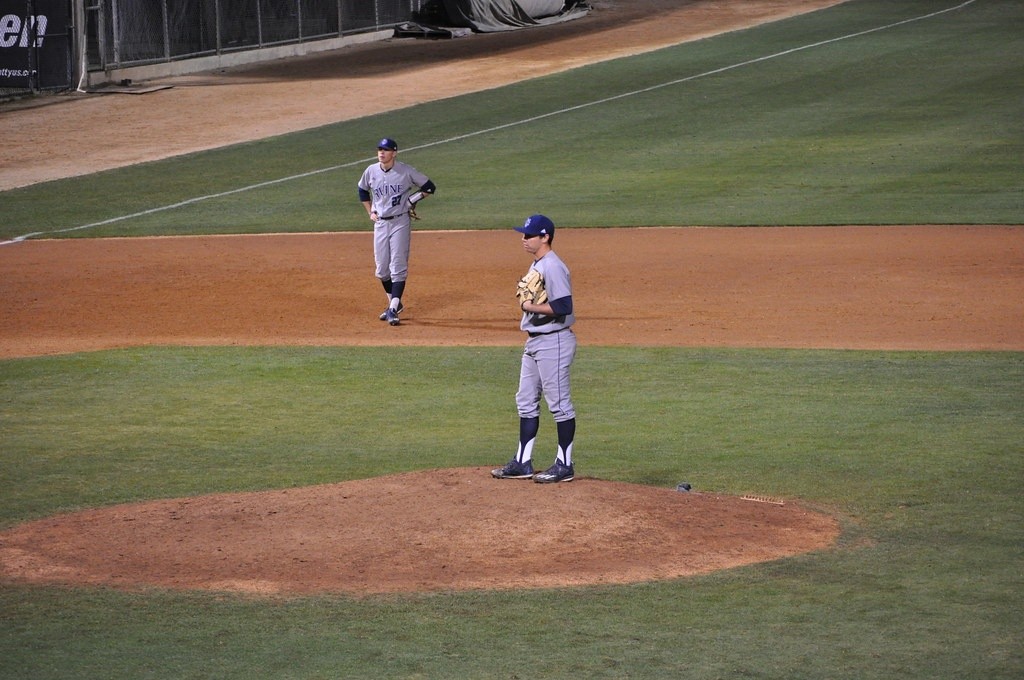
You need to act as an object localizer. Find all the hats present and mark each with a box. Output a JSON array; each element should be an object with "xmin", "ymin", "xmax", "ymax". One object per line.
[
  {"xmin": 377, "ymin": 137, "xmax": 397, "ymax": 151},
  {"xmin": 512, "ymin": 215, "xmax": 554, "ymax": 239}
]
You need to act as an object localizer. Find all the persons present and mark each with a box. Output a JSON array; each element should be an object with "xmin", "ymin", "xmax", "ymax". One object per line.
[
  {"xmin": 357, "ymin": 138, "xmax": 436, "ymax": 323},
  {"xmin": 489, "ymin": 215, "xmax": 577, "ymax": 483}
]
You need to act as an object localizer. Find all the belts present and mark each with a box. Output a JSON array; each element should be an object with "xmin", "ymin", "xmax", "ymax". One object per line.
[
  {"xmin": 380, "ymin": 213, "xmax": 403, "ymax": 220},
  {"xmin": 528, "ymin": 326, "xmax": 570, "ymax": 338}
]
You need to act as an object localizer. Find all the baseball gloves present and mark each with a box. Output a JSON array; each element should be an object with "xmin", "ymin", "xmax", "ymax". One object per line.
[
  {"xmin": 516, "ymin": 270, "xmax": 549, "ymax": 311},
  {"xmin": 408, "ymin": 206, "xmax": 422, "ymax": 223}
]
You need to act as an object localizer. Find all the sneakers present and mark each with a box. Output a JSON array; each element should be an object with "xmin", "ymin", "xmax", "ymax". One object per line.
[
  {"xmin": 532, "ymin": 458, "xmax": 574, "ymax": 483},
  {"xmin": 380, "ymin": 302, "xmax": 404, "ymax": 321},
  {"xmin": 490, "ymin": 454, "xmax": 534, "ymax": 478},
  {"xmin": 386, "ymin": 307, "xmax": 400, "ymax": 324}
]
